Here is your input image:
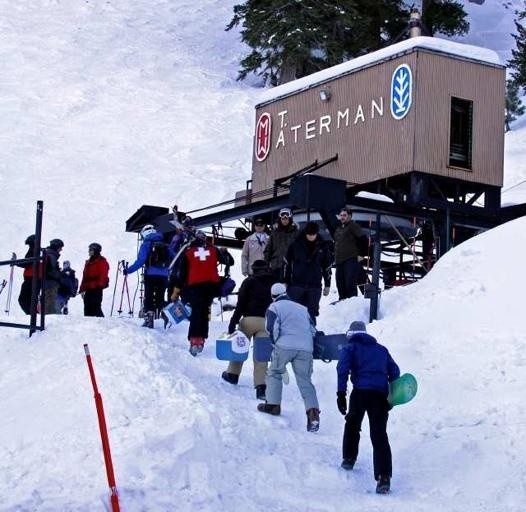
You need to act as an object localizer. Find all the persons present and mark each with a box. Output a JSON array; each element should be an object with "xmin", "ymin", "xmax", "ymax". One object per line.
[
  {"xmin": 123, "ymin": 208, "xmax": 371, "ymax": 352},
  {"xmin": 223, "ymin": 260, "xmax": 290, "ymax": 400},
  {"xmin": 18, "ymin": 233, "xmax": 78, "ymax": 315},
  {"xmin": 78, "ymin": 242, "xmax": 110, "ymax": 319},
  {"xmin": 257, "ymin": 282, "xmax": 323, "ymax": 432},
  {"xmin": 334, "ymin": 321, "xmax": 399, "ymax": 496}
]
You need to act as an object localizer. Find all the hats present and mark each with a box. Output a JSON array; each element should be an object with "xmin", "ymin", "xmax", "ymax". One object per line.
[
  {"xmin": 346, "ymin": 321, "xmax": 366, "ymax": 339},
  {"xmin": 253, "ymin": 216, "xmax": 266, "ymax": 226},
  {"xmin": 278, "ymin": 208, "xmax": 293, "ymax": 219},
  {"xmin": 270, "ymin": 282, "xmax": 286, "ymax": 300}
]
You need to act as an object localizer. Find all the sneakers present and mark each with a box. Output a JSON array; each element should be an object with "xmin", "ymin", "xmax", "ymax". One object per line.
[
  {"xmin": 254, "ymin": 384, "xmax": 266, "ymax": 400},
  {"xmin": 307, "ymin": 408, "xmax": 321, "ymax": 432},
  {"xmin": 375, "ymin": 472, "xmax": 390, "ymax": 494},
  {"xmin": 222, "ymin": 371, "xmax": 238, "ymax": 384},
  {"xmin": 257, "ymin": 400, "xmax": 280, "ymax": 415},
  {"xmin": 341, "ymin": 458, "xmax": 356, "ymax": 470}
]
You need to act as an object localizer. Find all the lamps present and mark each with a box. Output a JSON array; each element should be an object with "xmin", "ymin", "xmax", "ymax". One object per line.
[{"xmin": 319, "ymin": 86, "xmax": 331, "ymax": 102}]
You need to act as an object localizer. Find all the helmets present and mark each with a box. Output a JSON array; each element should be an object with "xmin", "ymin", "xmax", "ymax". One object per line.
[
  {"xmin": 88, "ymin": 243, "xmax": 101, "ymax": 257},
  {"xmin": 50, "ymin": 239, "xmax": 63, "ymax": 252},
  {"xmin": 140, "ymin": 224, "xmax": 157, "ymax": 238}
]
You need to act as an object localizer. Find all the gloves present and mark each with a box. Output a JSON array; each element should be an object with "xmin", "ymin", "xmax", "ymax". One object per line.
[
  {"xmin": 171, "ymin": 287, "xmax": 180, "ymax": 302},
  {"xmin": 323, "ymin": 286, "xmax": 330, "ymax": 296},
  {"xmin": 337, "ymin": 391, "xmax": 346, "ymax": 415},
  {"xmin": 229, "ymin": 321, "xmax": 236, "ymax": 334}
]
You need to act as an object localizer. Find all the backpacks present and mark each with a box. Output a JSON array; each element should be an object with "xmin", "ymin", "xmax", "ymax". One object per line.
[{"xmin": 145, "ymin": 239, "xmax": 172, "ymax": 268}]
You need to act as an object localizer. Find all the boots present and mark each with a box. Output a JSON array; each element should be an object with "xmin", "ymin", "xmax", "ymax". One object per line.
[
  {"xmin": 141, "ymin": 311, "xmax": 154, "ymax": 328},
  {"xmin": 196, "ymin": 335, "xmax": 205, "ymax": 353},
  {"xmin": 161, "ymin": 310, "xmax": 172, "ymax": 330},
  {"xmin": 190, "ymin": 336, "xmax": 198, "ymax": 356}
]
